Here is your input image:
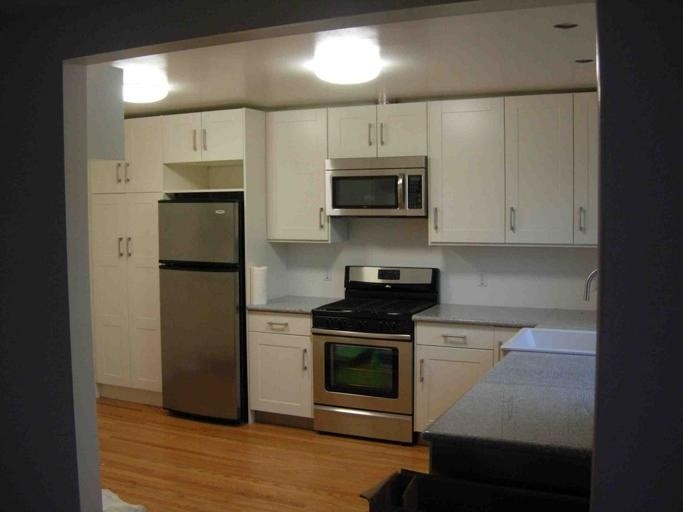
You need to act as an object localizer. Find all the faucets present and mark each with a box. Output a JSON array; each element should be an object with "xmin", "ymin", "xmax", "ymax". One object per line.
[{"xmin": 583, "ymin": 268, "xmax": 599, "ymax": 303}]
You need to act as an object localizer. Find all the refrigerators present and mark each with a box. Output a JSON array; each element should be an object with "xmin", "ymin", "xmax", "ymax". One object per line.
[{"xmin": 155, "ymin": 198, "xmax": 249, "ymax": 425}]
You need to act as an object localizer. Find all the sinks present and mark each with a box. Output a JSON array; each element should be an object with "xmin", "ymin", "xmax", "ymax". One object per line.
[{"xmin": 501, "ymin": 327, "xmax": 597, "ymax": 356}]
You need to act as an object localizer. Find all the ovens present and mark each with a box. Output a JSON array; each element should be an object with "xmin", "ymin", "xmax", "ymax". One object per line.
[{"xmin": 308, "ymin": 324, "xmax": 416, "ymax": 447}]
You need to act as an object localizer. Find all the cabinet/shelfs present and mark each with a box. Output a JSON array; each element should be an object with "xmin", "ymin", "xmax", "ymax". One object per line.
[
  {"xmin": 89, "ymin": 116, "xmax": 209, "ymax": 407},
  {"xmin": 246, "ymin": 311, "xmax": 314, "ymax": 419},
  {"xmin": 414, "ymin": 324, "xmax": 492, "ymax": 433},
  {"xmin": 266, "ymin": 108, "xmax": 351, "ymax": 246},
  {"xmin": 162, "ymin": 107, "xmax": 243, "ymax": 166},
  {"xmin": 493, "ymin": 327, "xmax": 525, "ymax": 365},
  {"xmin": 327, "ymin": 102, "xmax": 428, "ymax": 158},
  {"xmin": 427, "ymin": 92, "xmax": 599, "ymax": 249}
]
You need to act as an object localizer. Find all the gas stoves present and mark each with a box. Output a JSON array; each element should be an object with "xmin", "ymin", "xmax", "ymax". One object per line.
[{"xmin": 307, "ymin": 299, "xmax": 436, "ymax": 332}]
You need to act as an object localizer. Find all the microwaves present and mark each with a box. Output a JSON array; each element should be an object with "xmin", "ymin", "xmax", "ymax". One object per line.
[{"xmin": 322, "ymin": 155, "xmax": 426, "ymax": 217}]
